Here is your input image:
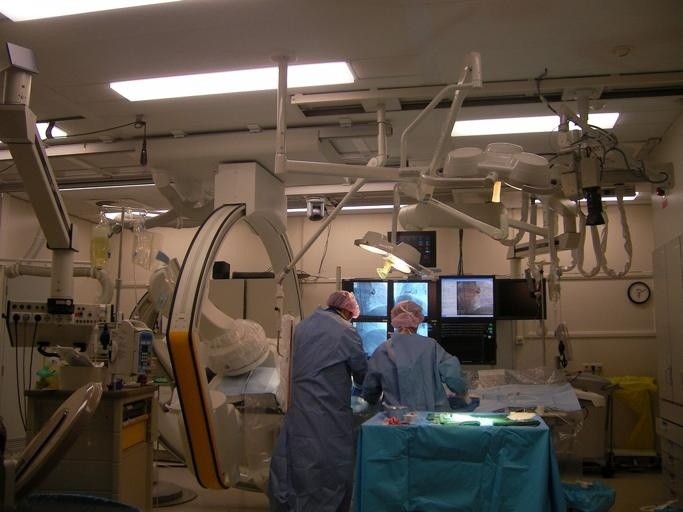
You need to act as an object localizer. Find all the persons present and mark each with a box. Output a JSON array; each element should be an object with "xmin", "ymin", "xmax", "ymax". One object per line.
[
  {"xmin": 361, "ymin": 300, "xmax": 470, "ymax": 414},
  {"xmin": 269, "ymin": 290, "xmax": 367, "ymax": 512}
]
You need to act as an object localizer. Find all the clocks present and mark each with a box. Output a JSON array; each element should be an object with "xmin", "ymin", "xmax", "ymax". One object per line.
[{"xmin": 627, "ymin": 281, "xmax": 652, "ymax": 305}]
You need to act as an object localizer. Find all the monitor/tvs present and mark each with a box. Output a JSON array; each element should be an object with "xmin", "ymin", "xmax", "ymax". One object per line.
[
  {"xmin": 438, "ymin": 319, "xmax": 498, "ymax": 366},
  {"xmin": 352, "ymin": 321, "xmax": 384, "ymax": 359},
  {"xmin": 392, "ymin": 281, "xmax": 430, "ymax": 316},
  {"xmin": 352, "ymin": 279, "xmax": 388, "ymax": 317},
  {"xmin": 438, "ymin": 275, "xmax": 494, "ymax": 318},
  {"xmin": 392, "ymin": 322, "xmax": 428, "ymax": 343},
  {"xmin": 387, "ymin": 230, "xmax": 436, "ymax": 268},
  {"xmin": 495, "ymin": 278, "xmax": 547, "ymax": 320}
]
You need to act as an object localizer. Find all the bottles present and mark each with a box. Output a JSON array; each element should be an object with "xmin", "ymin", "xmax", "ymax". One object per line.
[{"xmin": 90, "ymin": 226, "xmax": 109, "ymax": 271}]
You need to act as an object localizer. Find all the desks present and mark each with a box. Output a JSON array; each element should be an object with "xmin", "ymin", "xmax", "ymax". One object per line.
[{"xmin": 360, "ymin": 408, "xmax": 550, "ymax": 512}]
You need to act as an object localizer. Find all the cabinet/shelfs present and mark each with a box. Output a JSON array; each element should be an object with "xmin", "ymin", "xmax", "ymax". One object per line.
[
  {"xmin": 651, "ymin": 235, "xmax": 683, "ymax": 511},
  {"xmin": 24, "ymin": 382, "xmax": 160, "ymax": 511}
]
